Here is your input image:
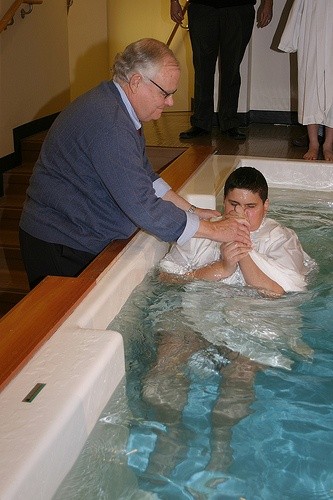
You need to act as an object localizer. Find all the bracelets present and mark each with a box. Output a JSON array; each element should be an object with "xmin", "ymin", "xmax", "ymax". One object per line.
[{"xmin": 188, "ymin": 205, "xmax": 197, "ymax": 214}]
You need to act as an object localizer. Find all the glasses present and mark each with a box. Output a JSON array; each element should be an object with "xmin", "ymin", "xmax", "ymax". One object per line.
[{"xmin": 148, "ymin": 78, "xmax": 177, "ymax": 99}]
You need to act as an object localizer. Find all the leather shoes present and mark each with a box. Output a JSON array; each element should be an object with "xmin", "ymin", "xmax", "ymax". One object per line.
[
  {"xmin": 180, "ymin": 126, "xmax": 210, "ymax": 140},
  {"xmin": 221, "ymin": 126, "xmax": 246, "ymax": 139}
]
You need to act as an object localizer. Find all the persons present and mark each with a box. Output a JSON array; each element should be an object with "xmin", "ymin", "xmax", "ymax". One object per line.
[
  {"xmin": 137, "ymin": 166, "xmax": 319, "ymax": 499},
  {"xmin": 18, "ymin": 38, "xmax": 253, "ymax": 290},
  {"xmin": 278, "ymin": 0, "xmax": 333, "ymax": 162},
  {"xmin": 170, "ymin": 0, "xmax": 273, "ymax": 139}
]
